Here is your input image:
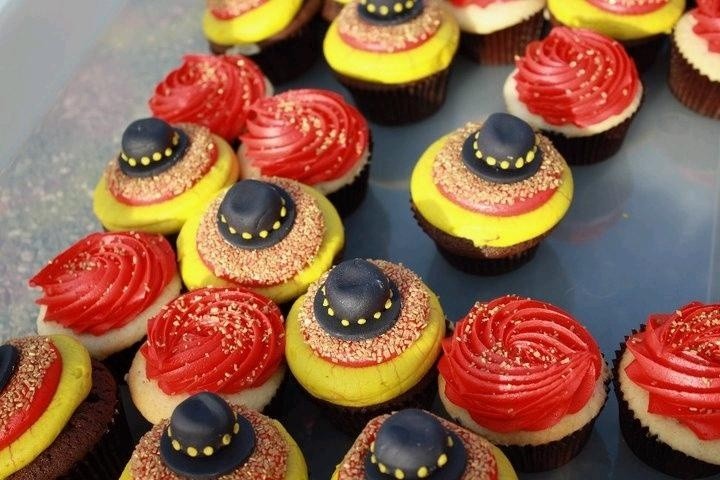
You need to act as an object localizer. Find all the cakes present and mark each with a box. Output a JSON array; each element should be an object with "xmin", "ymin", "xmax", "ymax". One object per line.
[
  {"xmin": 115, "ymin": 392, "xmax": 311, "ymax": 480},
  {"xmin": 329, "ymin": 408, "xmax": 517, "ymax": 480},
  {"xmin": 500, "ymin": 24, "xmax": 645, "ymax": 168},
  {"xmin": 322, "ymin": 1, "xmax": 461, "ymax": 130},
  {"xmin": 665, "ymin": 0, "xmax": 720, "ymax": 120},
  {"xmin": 232, "ymin": 85, "xmax": 375, "ymax": 220},
  {"xmin": 0, "ymin": 332, "xmax": 117, "ymax": 480},
  {"xmin": 545, "ymin": 1, "xmax": 685, "ymax": 83},
  {"xmin": 145, "ymin": 51, "xmax": 274, "ymax": 152},
  {"xmin": 610, "ymin": 302, "xmax": 720, "ymax": 474},
  {"xmin": 283, "ymin": 256, "xmax": 448, "ymax": 419},
  {"xmin": 409, "ymin": 114, "xmax": 573, "ymax": 278},
  {"xmin": 314, "ymin": 1, "xmax": 341, "ymax": 24},
  {"xmin": 121, "ymin": 284, "xmax": 286, "ymax": 427},
  {"xmin": 24, "ymin": 229, "xmax": 184, "ymax": 367},
  {"xmin": 174, "ymin": 175, "xmax": 347, "ymax": 304},
  {"xmin": 437, "ymin": 291, "xmax": 610, "ymax": 473},
  {"xmin": 89, "ymin": 117, "xmax": 240, "ymax": 247},
  {"xmin": 437, "ymin": 0, "xmax": 546, "ymax": 67},
  {"xmin": 199, "ymin": 0, "xmax": 320, "ymax": 87}
]
